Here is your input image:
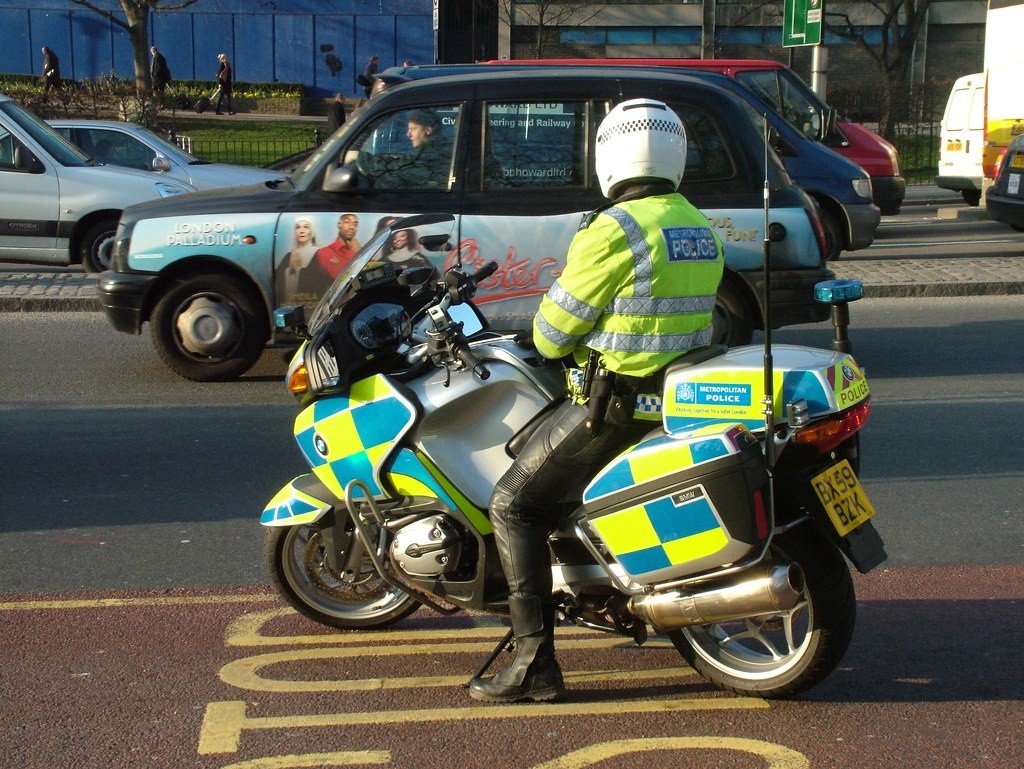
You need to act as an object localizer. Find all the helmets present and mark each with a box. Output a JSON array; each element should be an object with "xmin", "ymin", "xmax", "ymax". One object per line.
[{"xmin": 595, "ymin": 98, "xmax": 686, "ymax": 197}]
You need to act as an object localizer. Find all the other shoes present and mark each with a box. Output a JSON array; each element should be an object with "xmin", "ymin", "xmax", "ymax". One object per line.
[
  {"xmin": 229, "ymin": 111, "xmax": 236, "ymax": 115},
  {"xmin": 216, "ymin": 112, "xmax": 224, "ymax": 115}
]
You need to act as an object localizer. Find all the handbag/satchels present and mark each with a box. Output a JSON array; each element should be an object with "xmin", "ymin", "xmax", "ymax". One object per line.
[{"xmin": 356, "ymin": 74, "xmax": 368, "ymax": 87}]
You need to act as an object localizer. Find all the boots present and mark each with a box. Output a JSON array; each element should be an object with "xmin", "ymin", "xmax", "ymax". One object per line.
[{"xmin": 470, "ymin": 596, "xmax": 566, "ymax": 703}]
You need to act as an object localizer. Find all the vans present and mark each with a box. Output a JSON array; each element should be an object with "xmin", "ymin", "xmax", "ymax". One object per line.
[
  {"xmin": 935, "ymin": 72, "xmax": 988, "ymax": 206},
  {"xmin": 359, "ymin": 64, "xmax": 881, "ymax": 262},
  {"xmin": 477, "ymin": 58, "xmax": 906, "ymax": 216}
]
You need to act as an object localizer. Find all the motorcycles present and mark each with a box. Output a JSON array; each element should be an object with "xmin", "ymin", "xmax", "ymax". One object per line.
[{"xmin": 260, "ymin": 113, "xmax": 889, "ymax": 701}]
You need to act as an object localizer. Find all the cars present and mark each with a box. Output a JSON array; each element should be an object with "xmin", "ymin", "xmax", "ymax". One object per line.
[
  {"xmin": 0, "ymin": 94, "xmax": 200, "ymax": 273},
  {"xmin": 0, "ymin": 120, "xmax": 291, "ymax": 192},
  {"xmin": 97, "ymin": 68, "xmax": 836, "ymax": 385}
]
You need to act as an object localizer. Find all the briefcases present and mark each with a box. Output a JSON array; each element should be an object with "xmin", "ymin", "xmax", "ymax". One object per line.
[
  {"xmin": 173, "ymin": 93, "xmax": 192, "ymax": 111},
  {"xmin": 193, "ymin": 96, "xmax": 211, "ymax": 113}
]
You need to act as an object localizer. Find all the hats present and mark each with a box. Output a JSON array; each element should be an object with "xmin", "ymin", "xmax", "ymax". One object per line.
[{"xmin": 407, "ymin": 109, "xmax": 440, "ymax": 126}]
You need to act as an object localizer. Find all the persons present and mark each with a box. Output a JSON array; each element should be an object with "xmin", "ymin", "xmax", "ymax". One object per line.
[
  {"xmin": 271, "ymin": 214, "xmax": 420, "ymax": 307},
  {"xmin": 149, "ymin": 46, "xmax": 167, "ymax": 110},
  {"xmin": 403, "ymin": 61, "xmax": 415, "ymax": 66},
  {"xmin": 328, "ymin": 93, "xmax": 368, "ymax": 152},
  {"xmin": 216, "ymin": 54, "xmax": 236, "ymax": 115},
  {"xmin": 363, "ymin": 56, "xmax": 379, "ymax": 100},
  {"xmin": 343, "ymin": 109, "xmax": 454, "ymax": 189},
  {"xmin": 469, "ymin": 97, "xmax": 726, "ymax": 703},
  {"xmin": 96, "ymin": 140, "xmax": 133, "ymax": 167},
  {"xmin": 40, "ymin": 47, "xmax": 65, "ymax": 104}
]
